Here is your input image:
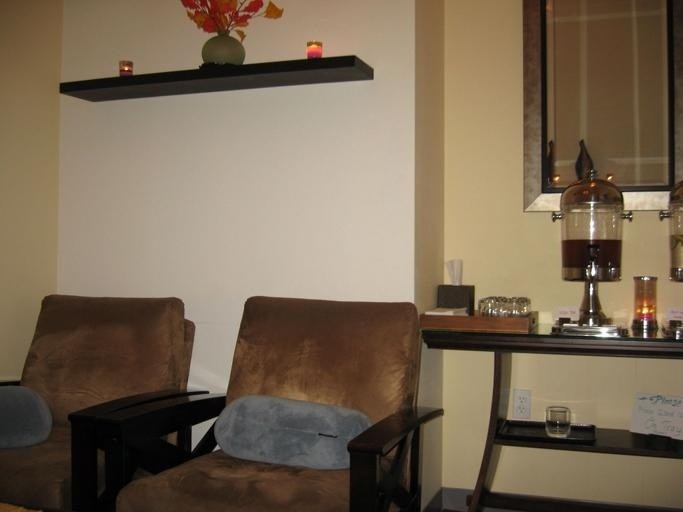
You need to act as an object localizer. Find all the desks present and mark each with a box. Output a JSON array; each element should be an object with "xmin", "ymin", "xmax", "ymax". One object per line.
[{"xmin": 423, "ymin": 326, "xmax": 683, "ymax": 512}]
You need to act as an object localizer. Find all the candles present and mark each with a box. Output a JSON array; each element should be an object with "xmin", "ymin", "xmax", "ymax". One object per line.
[
  {"xmin": 307, "ymin": 41, "xmax": 322, "ymax": 58},
  {"xmin": 119, "ymin": 60, "xmax": 133, "ymax": 75}
]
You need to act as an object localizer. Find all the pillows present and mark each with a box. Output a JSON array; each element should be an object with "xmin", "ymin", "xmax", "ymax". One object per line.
[
  {"xmin": 222, "ymin": 298, "xmax": 418, "ymax": 423},
  {"xmin": 0, "ymin": 385, "xmax": 53, "ymax": 449},
  {"xmin": 214, "ymin": 394, "xmax": 371, "ymax": 471},
  {"xmin": 21, "ymin": 294, "xmax": 184, "ymax": 424}
]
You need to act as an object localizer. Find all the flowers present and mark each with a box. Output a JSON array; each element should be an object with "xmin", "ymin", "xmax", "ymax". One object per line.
[{"xmin": 181, "ymin": 0, "xmax": 284, "ymax": 44}]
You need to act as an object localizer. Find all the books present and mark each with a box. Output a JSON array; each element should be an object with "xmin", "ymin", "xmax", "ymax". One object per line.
[{"xmin": 424, "ymin": 305, "xmax": 470, "ymax": 317}]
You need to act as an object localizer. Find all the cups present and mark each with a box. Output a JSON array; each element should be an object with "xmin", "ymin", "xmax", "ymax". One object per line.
[
  {"xmin": 477, "ymin": 296, "xmax": 531, "ymax": 317},
  {"xmin": 306, "ymin": 41, "xmax": 323, "ymax": 59},
  {"xmin": 118, "ymin": 60, "xmax": 134, "ymax": 76},
  {"xmin": 545, "ymin": 405, "xmax": 571, "ymax": 437}
]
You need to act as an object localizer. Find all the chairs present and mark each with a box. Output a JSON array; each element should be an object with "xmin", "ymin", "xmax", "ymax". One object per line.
[
  {"xmin": 94, "ymin": 296, "xmax": 444, "ymax": 512},
  {"xmin": 0, "ymin": 294, "xmax": 210, "ymax": 512}
]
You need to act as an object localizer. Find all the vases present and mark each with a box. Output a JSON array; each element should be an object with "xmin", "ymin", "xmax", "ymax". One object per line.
[{"xmin": 202, "ymin": 29, "xmax": 245, "ymax": 64}]
